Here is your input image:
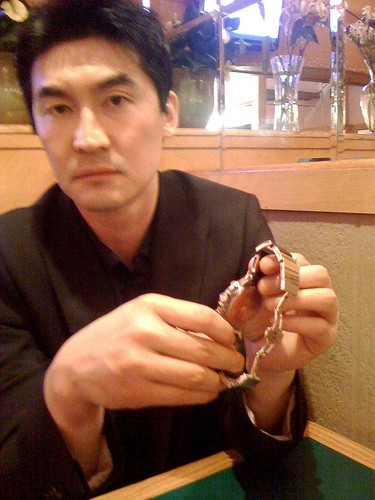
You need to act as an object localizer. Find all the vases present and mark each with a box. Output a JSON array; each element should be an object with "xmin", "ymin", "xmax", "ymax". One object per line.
[
  {"xmin": 271, "ymin": 54, "xmax": 304, "ymax": 132},
  {"xmin": 330, "ymin": 51, "xmax": 348, "ymax": 130},
  {"xmin": 171, "ymin": 66, "xmax": 217, "ymax": 128},
  {"xmin": 0, "ymin": 51, "xmax": 30, "ymax": 124}
]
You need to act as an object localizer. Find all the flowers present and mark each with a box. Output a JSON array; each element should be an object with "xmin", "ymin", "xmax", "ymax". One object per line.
[
  {"xmin": 346, "ymin": 4, "xmax": 375, "ymax": 59},
  {"xmin": 332, "ymin": 1, "xmax": 349, "ymax": 122},
  {"xmin": 276, "ymin": 0, "xmax": 327, "ymax": 56},
  {"xmin": 163, "ymin": 0, "xmax": 240, "ymax": 81},
  {"xmin": 358, "ymin": 57, "xmax": 375, "ymax": 133},
  {"xmin": 0, "ymin": 0, "xmax": 40, "ymax": 51}
]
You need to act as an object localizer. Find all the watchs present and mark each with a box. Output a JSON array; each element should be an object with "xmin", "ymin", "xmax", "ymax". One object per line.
[{"xmin": 211, "ymin": 240, "xmax": 299, "ymax": 390}]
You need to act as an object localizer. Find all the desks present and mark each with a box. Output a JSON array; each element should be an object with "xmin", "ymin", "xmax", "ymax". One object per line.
[{"xmin": 94, "ymin": 421, "xmax": 375, "ymax": 500}]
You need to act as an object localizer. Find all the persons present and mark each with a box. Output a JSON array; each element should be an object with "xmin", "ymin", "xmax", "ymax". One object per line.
[{"xmin": 1, "ymin": 0, "xmax": 341, "ymax": 500}]
